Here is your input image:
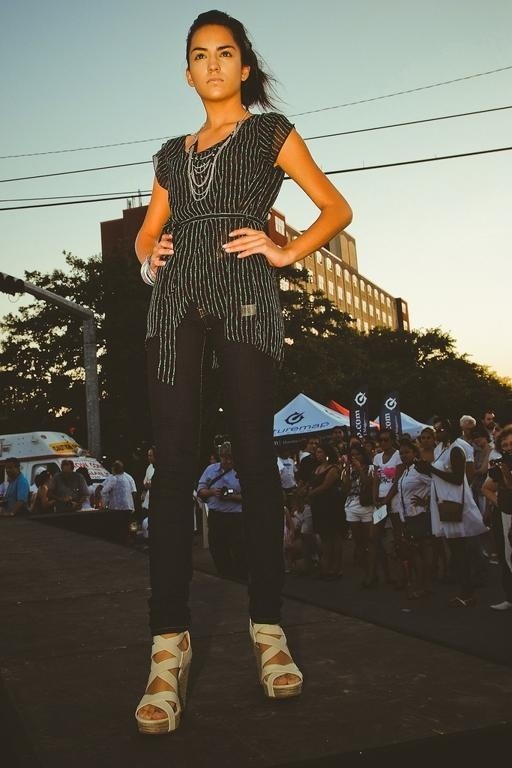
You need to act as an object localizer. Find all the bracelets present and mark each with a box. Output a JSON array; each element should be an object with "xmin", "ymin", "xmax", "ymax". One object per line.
[{"xmin": 140, "ymin": 255, "xmax": 157, "ymax": 287}]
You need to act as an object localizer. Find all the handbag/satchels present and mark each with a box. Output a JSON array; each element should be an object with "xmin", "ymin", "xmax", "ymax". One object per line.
[
  {"xmin": 372, "ymin": 505, "xmax": 389, "ymax": 526},
  {"xmin": 438, "ymin": 500, "xmax": 464, "ymax": 522},
  {"xmin": 404, "ymin": 511, "xmax": 431, "ymax": 538}
]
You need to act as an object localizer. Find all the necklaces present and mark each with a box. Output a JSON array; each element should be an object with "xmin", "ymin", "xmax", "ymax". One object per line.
[{"xmin": 187, "ymin": 110, "xmax": 248, "ymax": 202}]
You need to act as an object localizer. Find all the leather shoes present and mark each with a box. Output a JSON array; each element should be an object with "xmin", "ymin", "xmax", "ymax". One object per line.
[{"xmin": 490, "ymin": 600, "xmax": 511, "ymax": 611}]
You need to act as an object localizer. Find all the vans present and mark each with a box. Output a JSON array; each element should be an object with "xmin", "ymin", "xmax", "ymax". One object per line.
[{"xmin": 1, "ymin": 431, "xmax": 115, "ymax": 505}]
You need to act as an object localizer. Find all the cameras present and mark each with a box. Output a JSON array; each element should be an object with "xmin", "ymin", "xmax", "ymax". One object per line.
[{"xmin": 220, "ymin": 486, "xmax": 234, "ymax": 496}]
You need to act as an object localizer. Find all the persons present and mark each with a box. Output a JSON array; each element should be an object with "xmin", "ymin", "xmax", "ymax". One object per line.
[
  {"xmin": 276, "ymin": 408, "xmax": 512, "ymax": 609},
  {"xmin": 192, "ymin": 446, "xmax": 243, "ymax": 580},
  {"xmin": 133, "ymin": 11, "xmax": 354, "ymax": 740},
  {"xmin": 1, "ymin": 443, "xmax": 155, "ymax": 545}
]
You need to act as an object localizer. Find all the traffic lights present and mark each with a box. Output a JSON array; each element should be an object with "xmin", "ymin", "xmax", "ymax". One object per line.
[{"xmin": 0, "ymin": 273, "xmax": 24, "ymax": 298}]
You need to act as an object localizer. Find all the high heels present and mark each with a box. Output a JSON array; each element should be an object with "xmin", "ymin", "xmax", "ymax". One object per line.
[
  {"xmin": 136, "ymin": 631, "xmax": 192, "ymax": 734},
  {"xmin": 248, "ymin": 619, "xmax": 303, "ymax": 698}
]
